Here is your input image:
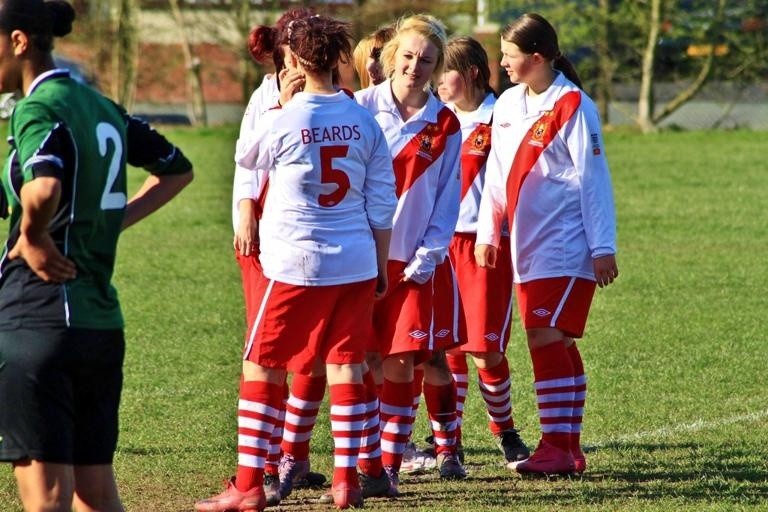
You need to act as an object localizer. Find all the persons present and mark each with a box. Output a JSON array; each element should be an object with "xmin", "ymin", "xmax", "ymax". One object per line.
[
  {"xmin": 0, "ymin": 0, "xmax": 194, "ymax": 512},
  {"xmin": 191, "ymin": 9, "xmax": 531, "ymax": 511},
  {"xmin": 474, "ymin": 12, "xmax": 620, "ymax": 480}
]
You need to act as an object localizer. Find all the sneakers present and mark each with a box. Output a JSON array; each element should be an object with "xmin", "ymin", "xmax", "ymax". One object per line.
[
  {"xmin": 516, "ymin": 440, "xmax": 585, "ymax": 473},
  {"xmin": 494, "ymin": 429, "xmax": 529, "ymax": 462},
  {"xmin": 193, "ymin": 454, "xmax": 326, "ymax": 512},
  {"xmin": 319, "ymin": 443, "xmax": 466, "ymax": 509}
]
